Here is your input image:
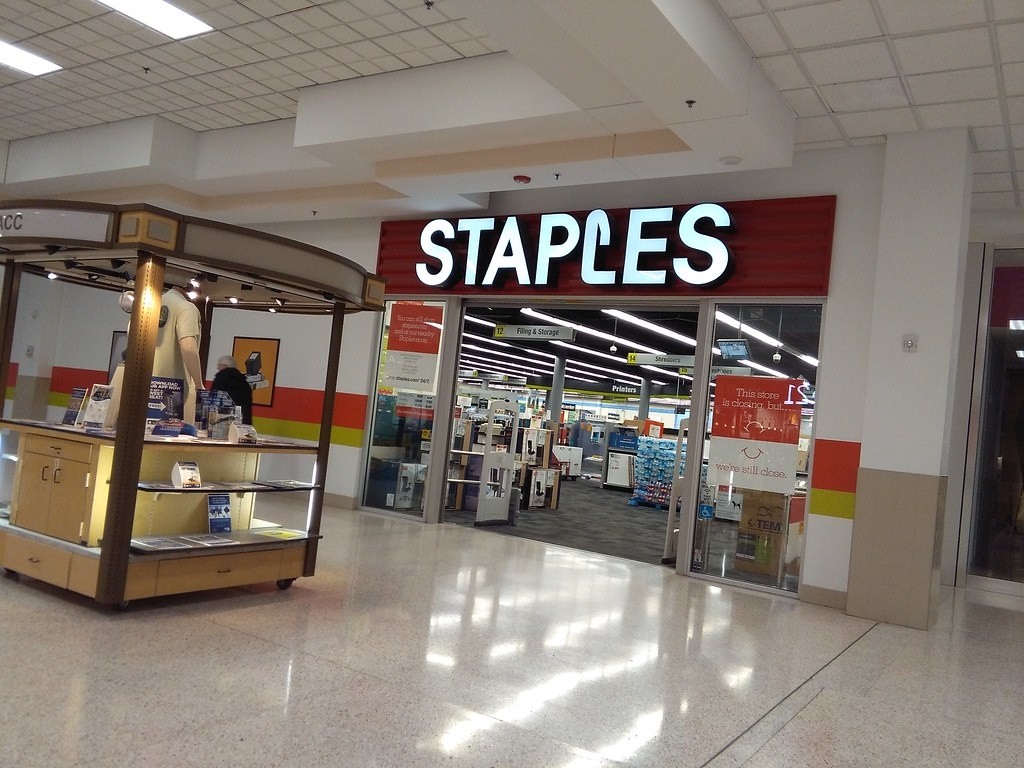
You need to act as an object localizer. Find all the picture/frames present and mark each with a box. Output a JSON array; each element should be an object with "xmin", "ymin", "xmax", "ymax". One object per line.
[{"xmin": 231, "ymin": 336, "xmax": 280, "ymax": 407}]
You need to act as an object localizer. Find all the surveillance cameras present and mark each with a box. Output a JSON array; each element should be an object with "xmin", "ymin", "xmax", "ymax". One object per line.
[
  {"xmin": 773, "ymin": 353, "xmax": 781, "ymax": 363},
  {"xmin": 610, "ymin": 346, "xmax": 617, "ymax": 354}
]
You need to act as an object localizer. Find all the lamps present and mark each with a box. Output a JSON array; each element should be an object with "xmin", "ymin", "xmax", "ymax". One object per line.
[
  {"xmin": 275, "ymin": 299, "xmax": 285, "ymax": 306},
  {"xmin": 458, "ymin": 307, "xmax": 819, "ymax": 383}
]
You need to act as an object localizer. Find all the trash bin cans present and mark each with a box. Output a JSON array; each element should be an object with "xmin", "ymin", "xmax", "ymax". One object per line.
[{"xmin": 691, "ymin": 503, "xmax": 714, "ymax": 571}]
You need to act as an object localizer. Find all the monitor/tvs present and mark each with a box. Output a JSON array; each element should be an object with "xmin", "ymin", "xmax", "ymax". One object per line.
[{"xmin": 717, "ymin": 339, "xmax": 752, "ymax": 360}]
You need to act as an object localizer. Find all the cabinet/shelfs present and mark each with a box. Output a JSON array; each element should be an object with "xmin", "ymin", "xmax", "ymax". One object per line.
[
  {"xmin": 600, "ymin": 423, "xmax": 639, "ymax": 489},
  {"xmin": 0, "ymin": 417, "xmax": 321, "ymax": 612}
]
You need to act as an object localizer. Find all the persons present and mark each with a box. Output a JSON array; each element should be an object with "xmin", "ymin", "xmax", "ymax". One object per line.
[
  {"xmin": 210, "ymin": 355, "xmax": 252, "ymax": 426},
  {"xmin": 152, "ymin": 284, "xmax": 206, "ymax": 425}
]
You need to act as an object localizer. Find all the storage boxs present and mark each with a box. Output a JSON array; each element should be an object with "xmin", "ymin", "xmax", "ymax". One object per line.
[{"xmin": 735, "ymin": 490, "xmax": 807, "ymax": 577}]
